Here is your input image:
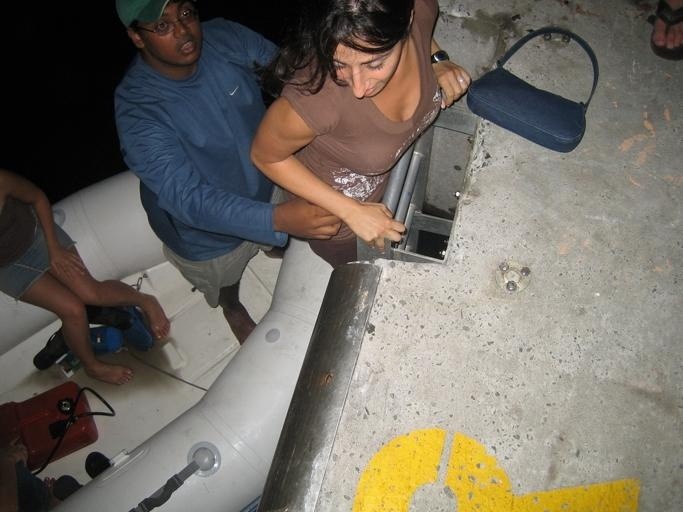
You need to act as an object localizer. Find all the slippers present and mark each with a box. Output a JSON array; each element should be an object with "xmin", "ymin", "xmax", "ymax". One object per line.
[
  {"xmin": 85, "ymin": 452, "xmax": 111, "ymax": 479},
  {"xmin": 53, "ymin": 475, "xmax": 81, "ymax": 500},
  {"xmin": 33, "ymin": 305, "xmax": 153, "ymax": 371}
]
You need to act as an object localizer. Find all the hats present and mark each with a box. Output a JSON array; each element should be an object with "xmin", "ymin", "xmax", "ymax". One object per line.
[{"xmin": 116, "ymin": 0, "xmax": 170, "ymax": 28}]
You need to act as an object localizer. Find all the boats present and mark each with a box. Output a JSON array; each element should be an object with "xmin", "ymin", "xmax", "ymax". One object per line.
[{"xmin": 0, "ymin": 152, "xmax": 332, "ymax": 512}]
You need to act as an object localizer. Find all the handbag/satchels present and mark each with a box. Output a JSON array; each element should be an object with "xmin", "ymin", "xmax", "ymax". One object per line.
[{"xmin": 466, "ymin": 67, "xmax": 586, "ymax": 153}]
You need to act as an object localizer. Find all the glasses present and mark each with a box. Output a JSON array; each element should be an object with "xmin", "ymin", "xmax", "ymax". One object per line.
[{"xmin": 137, "ymin": 7, "xmax": 196, "ymax": 35}]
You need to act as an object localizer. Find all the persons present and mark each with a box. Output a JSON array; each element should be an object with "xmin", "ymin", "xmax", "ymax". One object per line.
[
  {"xmin": 0, "ymin": 167, "xmax": 171, "ymax": 386},
  {"xmin": 0, "ymin": 435, "xmax": 61, "ymax": 512},
  {"xmin": 248, "ymin": 1, "xmax": 473, "ymax": 269},
  {"xmin": 113, "ymin": 0, "xmax": 343, "ymax": 345}
]
